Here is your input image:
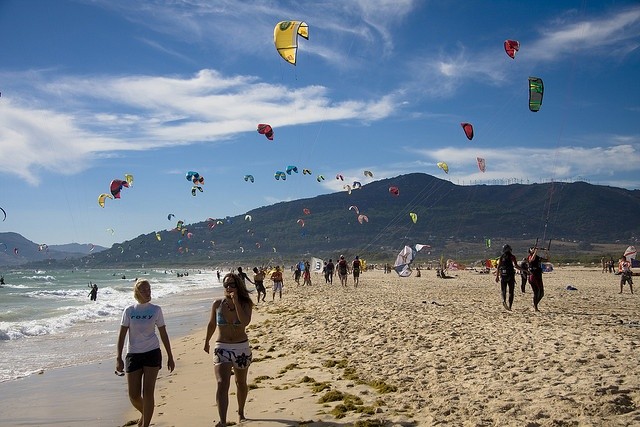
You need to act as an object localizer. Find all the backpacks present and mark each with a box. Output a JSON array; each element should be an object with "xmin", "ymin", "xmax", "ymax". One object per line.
[{"xmin": 500, "ymin": 255, "xmax": 515, "ymax": 284}]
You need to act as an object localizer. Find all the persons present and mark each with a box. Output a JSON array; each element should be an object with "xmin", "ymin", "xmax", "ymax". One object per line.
[
  {"xmin": 253, "ymin": 268, "xmax": 267, "ymax": 303},
  {"xmin": 338, "ymin": 255, "xmax": 350, "ymax": 286},
  {"xmin": 600, "ymin": 256, "xmax": 608, "ymax": 273},
  {"xmin": 270, "ymin": 266, "xmax": 283, "ymax": 300},
  {"xmin": 326, "ymin": 259, "xmax": 334, "ymax": 285},
  {"xmin": 116, "ymin": 279, "xmax": 175, "ymax": 427},
  {"xmin": 608, "ymin": 256, "xmax": 615, "ymax": 273},
  {"xmin": 301, "ymin": 259, "xmax": 311, "ymax": 286},
  {"xmin": 204, "ymin": 273, "xmax": 254, "ymax": 427},
  {"xmin": 351, "ymin": 256, "xmax": 362, "ymax": 287},
  {"xmin": 618, "ymin": 256, "xmax": 634, "ymax": 294},
  {"xmin": 527, "ymin": 246, "xmax": 549, "ymax": 312},
  {"xmin": 322, "ymin": 262, "xmax": 330, "ymax": 283},
  {"xmin": 292, "ymin": 267, "xmax": 300, "ymax": 286},
  {"xmin": 495, "ymin": 244, "xmax": 529, "ymax": 311},
  {"xmin": 521, "ymin": 258, "xmax": 529, "ymax": 292},
  {"xmin": 87, "ymin": 281, "xmax": 98, "ymax": 301},
  {"xmin": 415, "ymin": 268, "xmax": 420, "ymax": 277},
  {"xmin": 237, "ymin": 267, "xmax": 255, "ymax": 295},
  {"xmin": 335, "ymin": 260, "xmax": 341, "ymax": 278}
]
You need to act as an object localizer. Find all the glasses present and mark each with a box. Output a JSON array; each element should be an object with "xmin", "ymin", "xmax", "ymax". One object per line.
[
  {"xmin": 114, "ymin": 371, "xmax": 125, "ymax": 377},
  {"xmin": 223, "ymin": 282, "xmax": 237, "ymax": 289}
]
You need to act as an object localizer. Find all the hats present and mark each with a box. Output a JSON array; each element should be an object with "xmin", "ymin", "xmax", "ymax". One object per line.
[
  {"xmin": 329, "ymin": 259, "xmax": 332, "ymax": 262},
  {"xmin": 501, "ymin": 244, "xmax": 512, "ymax": 253},
  {"xmin": 355, "ymin": 256, "xmax": 359, "ymax": 259},
  {"xmin": 522, "ymin": 258, "xmax": 526, "ymax": 260},
  {"xmin": 305, "ymin": 260, "xmax": 310, "ymax": 263},
  {"xmin": 340, "ymin": 255, "xmax": 344, "ymax": 259}
]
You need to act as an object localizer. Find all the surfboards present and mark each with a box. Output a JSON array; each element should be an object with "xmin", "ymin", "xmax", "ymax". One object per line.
[{"xmin": 540, "ymin": 262, "xmax": 553, "ymax": 272}]
